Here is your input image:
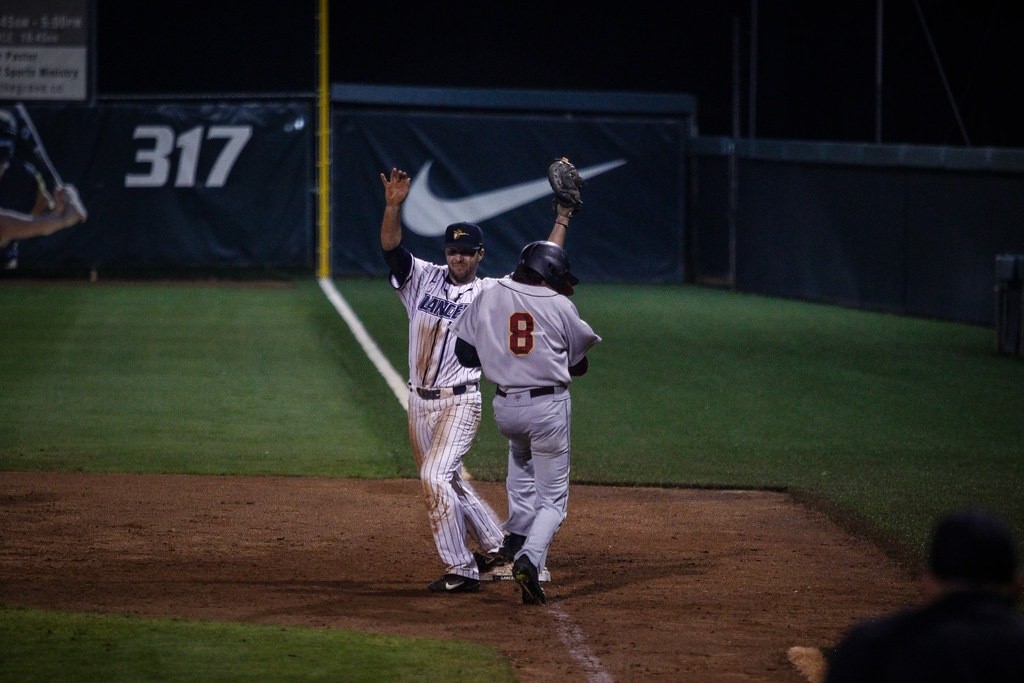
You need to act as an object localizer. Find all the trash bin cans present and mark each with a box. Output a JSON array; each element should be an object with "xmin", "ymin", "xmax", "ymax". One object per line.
[{"xmin": 994, "ymin": 252, "xmax": 1024, "ymax": 357}]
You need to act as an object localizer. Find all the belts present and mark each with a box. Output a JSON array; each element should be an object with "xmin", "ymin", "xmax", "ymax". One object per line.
[
  {"xmin": 409, "ymin": 382, "xmax": 480, "ymax": 400},
  {"xmin": 497, "ymin": 384, "xmax": 568, "ymax": 400}
]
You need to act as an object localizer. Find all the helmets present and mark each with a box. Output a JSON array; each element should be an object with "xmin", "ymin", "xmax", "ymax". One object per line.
[{"xmin": 513, "ymin": 240, "xmax": 578, "ymax": 296}]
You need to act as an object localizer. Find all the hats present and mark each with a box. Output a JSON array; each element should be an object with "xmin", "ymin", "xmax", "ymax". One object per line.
[
  {"xmin": 442, "ymin": 221, "xmax": 483, "ymax": 251},
  {"xmin": 928, "ymin": 510, "xmax": 1019, "ymax": 586}
]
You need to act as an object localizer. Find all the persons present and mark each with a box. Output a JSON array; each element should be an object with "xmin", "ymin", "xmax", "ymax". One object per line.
[
  {"xmin": 376, "ymin": 155, "xmax": 588, "ymax": 597},
  {"xmin": 0, "ymin": 108, "xmax": 84, "ymax": 253},
  {"xmin": 813, "ymin": 515, "xmax": 1023, "ymax": 683},
  {"xmin": 455, "ymin": 238, "xmax": 600, "ymax": 602}
]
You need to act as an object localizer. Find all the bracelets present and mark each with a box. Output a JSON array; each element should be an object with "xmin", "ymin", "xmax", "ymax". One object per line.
[{"xmin": 553, "ymin": 213, "xmax": 572, "ymax": 230}]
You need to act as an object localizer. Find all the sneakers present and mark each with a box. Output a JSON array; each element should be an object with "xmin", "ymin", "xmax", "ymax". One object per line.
[
  {"xmin": 513, "ymin": 554, "xmax": 546, "ymax": 605},
  {"xmin": 428, "ymin": 574, "xmax": 480, "ymax": 596},
  {"xmin": 499, "ymin": 533, "xmax": 526, "ymax": 562},
  {"xmin": 473, "ymin": 552, "xmax": 499, "ymax": 574}
]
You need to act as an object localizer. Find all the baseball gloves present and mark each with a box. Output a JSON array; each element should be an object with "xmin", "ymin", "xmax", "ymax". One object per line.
[{"xmin": 548, "ymin": 157, "xmax": 590, "ymax": 212}]
